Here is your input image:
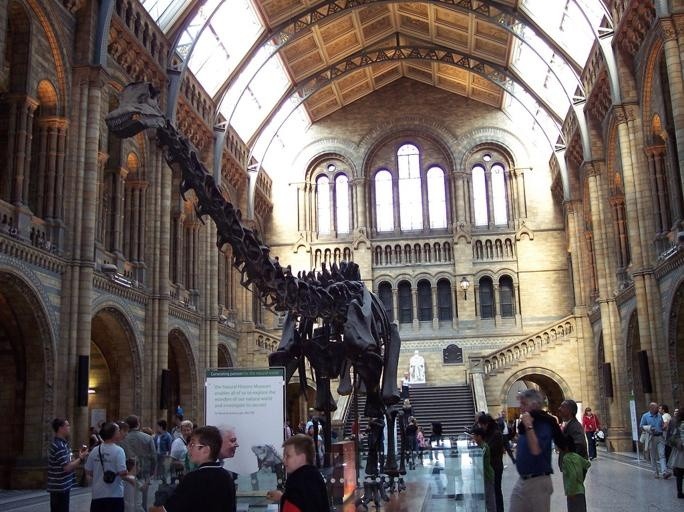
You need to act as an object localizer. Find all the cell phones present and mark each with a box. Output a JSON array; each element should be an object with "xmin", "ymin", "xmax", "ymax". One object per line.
[{"xmin": 82, "ymin": 445, "xmax": 87, "ymax": 453}]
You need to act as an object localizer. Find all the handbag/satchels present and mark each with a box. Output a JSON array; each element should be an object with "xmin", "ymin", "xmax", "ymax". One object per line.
[
  {"xmin": 103, "ymin": 470, "xmax": 116, "ymax": 484},
  {"xmin": 669, "ymin": 435, "xmax": 684, "ymax": 449},
  {"xmin": 594, "ymin": 431, "xmax": 603, "ymax": 441}
]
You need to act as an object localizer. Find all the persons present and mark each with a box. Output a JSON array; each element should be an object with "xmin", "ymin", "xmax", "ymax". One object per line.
[
  {"xmin": 400, "ymin": 372, "xmax": 410, "ymax": 399},
  {"xmin": 406, "ymin": 408, "xmax": 600, "ymax": 470},
  {"xmin": 351, "ymin": 414, "xmax": 364, "ymax": 469},
  {"xmin": 370, "ymin": 415, "xmax": 385, "ymax": 473},
  {"xmin": 46, "ymin": 407, "xmax": 333, "ymax": 512},
  {"xmin": 409, "ymin": 349, "xmax": 425, "ymax": 381},
  {"xmin": 562, "ymin": 433, "xmax": 591, "ymax": 512},
  {"xmin": 558, "ymin": 399, "xmax": 588, "ymax": 481},
  {"xmin": 639, "ymin": 402, "xmax": 684, "ymax": 499},
  {"xmin": 476, "ymin": 414, "xmax": 505, "ymax": 512},
  {"xmin": 401, "ymin": 398, "xmax": 414, "ymax": 427},
  {"xmin": 508, "ymin": 388, "xmax": 554, "ymax": 512}
]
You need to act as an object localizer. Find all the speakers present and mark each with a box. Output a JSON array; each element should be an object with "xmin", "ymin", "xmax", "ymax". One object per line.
[
  {"xmin": 602, "ymin": 362, "xmax": 613, "ymax": 397},
  {"xmin": 161, "ymin": 369, "xmax": 171, "ymax": 409},
  {"xmin": 637, "ymin": 350, "xmax": 652, "ymax": 394},
  {"xmin": 78, "ymin": 355, "xmax": 90, "ymax": 407}
]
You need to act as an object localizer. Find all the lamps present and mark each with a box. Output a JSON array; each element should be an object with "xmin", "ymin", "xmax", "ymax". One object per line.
[{"xmin": 459, "ymin": 275, "xmax": 471, "ymax": 300}]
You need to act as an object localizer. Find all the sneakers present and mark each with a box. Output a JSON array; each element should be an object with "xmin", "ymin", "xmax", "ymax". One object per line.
[{"xmin": 664, "ymin": 471, "xmax": 672, "ymax": 478}]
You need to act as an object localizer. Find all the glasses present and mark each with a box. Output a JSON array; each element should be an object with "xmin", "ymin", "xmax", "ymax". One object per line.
[{"xmin": 190, "ymin": 443, "xmax": 205, "ymax": 449}]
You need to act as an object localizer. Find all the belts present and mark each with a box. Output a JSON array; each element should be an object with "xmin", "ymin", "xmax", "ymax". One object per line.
[{"xmin": 522, "ymin": 472, "xmax": 550, "ymax": 480}]
